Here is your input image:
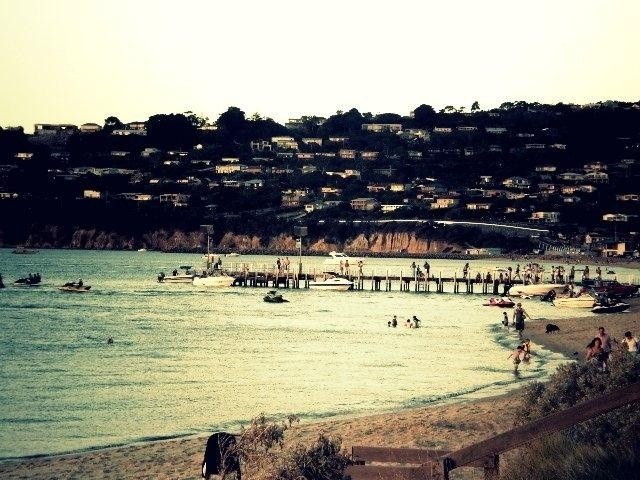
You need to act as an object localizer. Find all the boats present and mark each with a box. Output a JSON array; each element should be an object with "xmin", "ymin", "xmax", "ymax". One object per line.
[
  {"xmin": 14, "ymin": 272, "xmax": 44, "ymax": 287},
  {"xmin": 192, "ymin": 267, "xmax": 236, "ymax": 288},
  {"xmin": 483, "ymin": 295, "xmax": 514, "ymax": 308},
  {"xmin": 508, "ymin": 281, "xmax": 563, "ymax": 297},
  {"xmin": 591, "ymin": 299, "xmax": 630, "ymax": 315},
  {"xmin": 308, "ymin": 272, "xmax": 354, "ymax": 292},
  {"xmin": 552, "ymin": 294, "xmax": 595, "ymax": 309},
  {"xmin": 324, "ymin": 251, "xmax": 365, "ymax": 265},
  {"xmin": 58, "ymin": 281, "xmax": 91, "ymax": 293}
]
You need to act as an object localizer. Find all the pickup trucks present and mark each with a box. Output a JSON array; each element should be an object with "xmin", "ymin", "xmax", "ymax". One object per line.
[{"xmin": 583, "ymin": 279, "xmax": 638, "ymax": 299}]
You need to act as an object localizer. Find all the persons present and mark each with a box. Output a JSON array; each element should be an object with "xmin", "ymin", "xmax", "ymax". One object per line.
[
  {"xmin": 75, "ymin": 278, "xmax": 83, "ymax": 289},
  {"xmin": 410, "ymin": 262, "xmax": 430, "ymax": 278},
  {"xmin": 413, "ymin": 315, "xmax": 421, "ymax": 328},
  {"xmin": 583, "ymin": 265, "xmax": 602, "ymax": 280},
  {"xmin": 512, "ymin": 302, "xmax": 532, "ymax": 337},
  {"xmin": 584, "ymin": 337, "xmax": 604, "ymax": 363},
  {"xmin": 506, "ymin": 345, "xmax": 523, "ymax": 371},
  {"xmin": 522, "ymin": 339, "xmax": 531, "ymax": 362},
  {"xmin": 463, "ymin": 263, "xmax": 469, "ymax": 280},
  {"xmin": 551, "ymin": 266, "xmax": 575, "ymax": 283},
  {"xmin": 404, "ymin": 319, "xmax": 411, "ymax": 328},
  {"xmin": 392, "ymin": 315, "xmax": 398, "ymax": 327},
  {"xmin": 594, "ymin": 327, "xmax": 611, "ymax": 351},
  {"xmin": 277, "ymin": 258, "xmax": 290, "ymax": 272},
  {"xmin": 499, "ymin": 267, "xmax": 512, "ymax": 282},
  {"xmin": 501, "ymin": 312, "xmax": 510, "ymax": 331},
  {"xmin": 476, "ymin": 272, "xmax": 490, "ymax": 282},
  {"xmin": 513, "ymin": 264, "xmax": 540, "ymax": 282},
  {"xmin": 340, "ymin": 260, "xmax": 363, "ymax": 276},
  {"xmin": 621, "ymin": 331, "xmax": 640, "ymax": 355}
]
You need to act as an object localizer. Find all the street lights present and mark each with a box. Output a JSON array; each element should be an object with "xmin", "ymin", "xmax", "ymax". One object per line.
[
  {"xmin": 201, "ymin": 225, "xmax": 215, "ymax": 268},
  {"xmin": 294, "ymin": 225, "xmax": 308, "ymax": 274}
]
